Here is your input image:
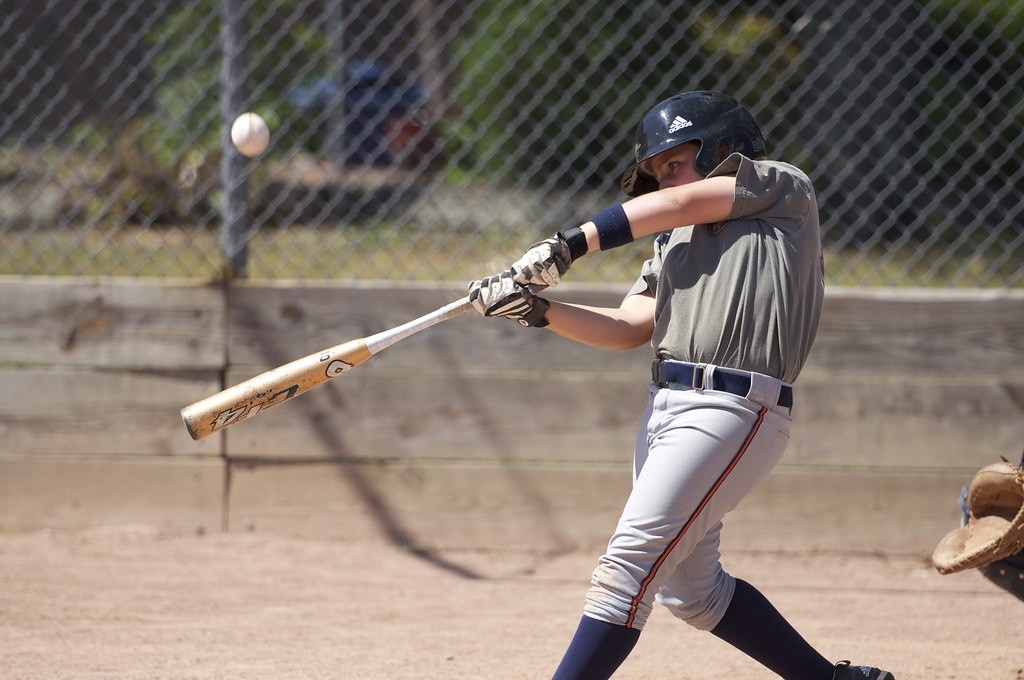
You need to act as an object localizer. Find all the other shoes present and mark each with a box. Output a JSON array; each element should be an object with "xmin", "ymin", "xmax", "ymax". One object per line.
[{"xmin": 833, "ymin": 660, "xmax": 894, "ymax": 680}]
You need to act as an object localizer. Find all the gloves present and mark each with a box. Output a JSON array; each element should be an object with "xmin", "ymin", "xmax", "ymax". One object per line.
[
  {"xmin": 509, "ymin": 231, "xmax": 571, "ymax": 298},
  {"xmin": 468, "ymin": 270, "xmax": 551, "ymax": 327}
]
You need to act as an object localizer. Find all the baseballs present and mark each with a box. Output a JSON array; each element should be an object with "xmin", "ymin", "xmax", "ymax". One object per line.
[{"xmin": 229, "ymin": 113, "xmax": 270, "ymax": 158}]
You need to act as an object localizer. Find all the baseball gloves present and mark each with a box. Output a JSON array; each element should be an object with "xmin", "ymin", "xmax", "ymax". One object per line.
[{"xmin": 930, "ymin": 454, "xmax": 1024, "ymax": 574}]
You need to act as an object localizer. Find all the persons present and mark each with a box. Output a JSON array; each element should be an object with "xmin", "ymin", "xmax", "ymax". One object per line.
[{"xmin": 469, "ymin": 89, "xmax": 897, "ymax": 680}]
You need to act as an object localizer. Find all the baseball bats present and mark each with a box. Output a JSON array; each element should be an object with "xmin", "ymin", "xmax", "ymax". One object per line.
[{"xmin": 182, "ymin": 297, "xmax": 476, "ymax": 442}]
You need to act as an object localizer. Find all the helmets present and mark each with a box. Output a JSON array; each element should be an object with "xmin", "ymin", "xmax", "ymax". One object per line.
[{"xmin": 621, "ymin": 91, "xmax": 768, "ymax": 197}]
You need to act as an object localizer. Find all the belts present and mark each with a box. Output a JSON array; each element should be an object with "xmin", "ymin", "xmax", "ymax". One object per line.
[{"xmin": 651, "ymin": 361, "xmax": 793, "ymax": 407}]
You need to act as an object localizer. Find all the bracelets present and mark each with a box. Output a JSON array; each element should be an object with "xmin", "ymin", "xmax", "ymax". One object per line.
[{"xmin": 589, "ymin": 203, "xmax": 635, "ymax": 251}]
